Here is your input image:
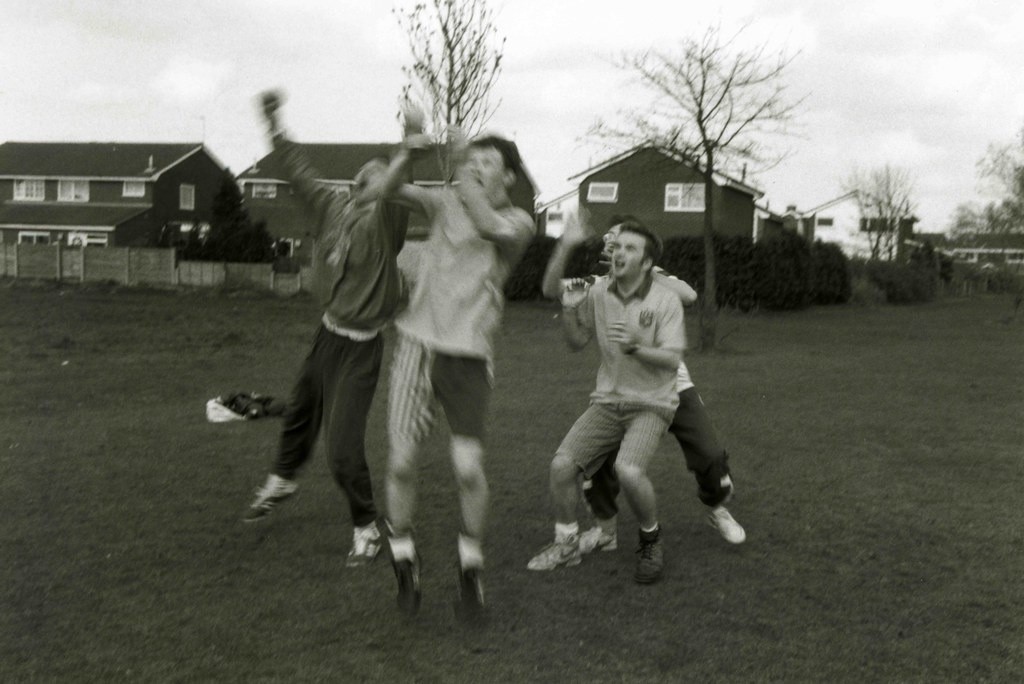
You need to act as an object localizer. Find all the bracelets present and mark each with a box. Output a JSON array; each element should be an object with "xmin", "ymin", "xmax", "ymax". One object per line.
[{"xmin": 626, "ymin": 344, "xmax": 639, "ymax": 357}]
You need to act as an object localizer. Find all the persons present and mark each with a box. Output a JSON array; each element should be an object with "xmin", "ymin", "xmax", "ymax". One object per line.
[
  {"xmin": 240, "ymin": 89, "xmax": 426, "ymax": 568},
  {"xmin": 525, "ymin": 221, "xmax": 680, "ymax": 584},
  {"xmin": 541, "ymin": 206, "xmax": 748, "ymax": 556},
  {"xmin": 356, "ymin": 134, "xmax": 539, "ymax": 615}
]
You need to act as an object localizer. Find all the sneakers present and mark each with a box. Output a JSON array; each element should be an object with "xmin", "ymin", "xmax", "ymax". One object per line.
[
  {"xmin": 242, "ymin": 475, "xmax": 300, "ymax": 523},
  {"xmin": 575, "ymin": 524, "xmax": 619, "ymax": 557},
  {"xmin": 630, "ymin": 524, "xmax": 665, "ymax": 583},
  {"xmin": 706, "ymin": 506, "xmax": 747, "ymax": 545},
  {"xmin": 345, "ymin": 523, "xmax": 385, "ymax": 570},
  {"xmin": 456, "ymin": 558, "xmax": 488, "ymax": 612},
  {"xmin": 390, "ymin": 551, "xmax": 423, "ymax": 617},
  {"xmin": 527, "ymin": 533, "xmax": 583, "ymax": 573}
]
